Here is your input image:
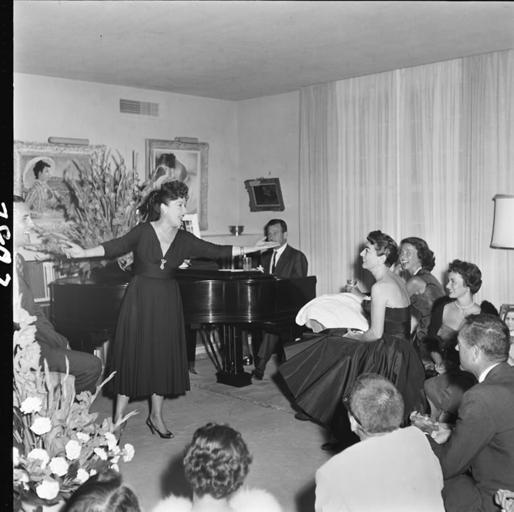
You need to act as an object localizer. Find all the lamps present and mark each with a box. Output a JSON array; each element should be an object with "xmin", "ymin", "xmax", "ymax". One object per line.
[{"xmin": 489, "ymin": 193, "xmax": 514, "ymax": 329}]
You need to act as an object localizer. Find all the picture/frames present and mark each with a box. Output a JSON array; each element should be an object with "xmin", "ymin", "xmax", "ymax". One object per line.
[
  {"xmin": 10, "ymin": 140, "xmax": 108, "ymax": 219},
  {"xmin": 145, "ymin": 138, "xmax": 209, "ymax": 231},
  {"xmin": 244, "ymin": 177, "xmax": 285, "ymax": 213}
]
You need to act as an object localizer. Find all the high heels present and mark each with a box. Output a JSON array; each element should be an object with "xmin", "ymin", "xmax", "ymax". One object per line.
[
  {"xmin": 113, "ymin": 420, "xmax": 127, "ymax": 445},
  {"xmin": 146, "ymin": 413, "xmax": 174, "ymax": 439}
]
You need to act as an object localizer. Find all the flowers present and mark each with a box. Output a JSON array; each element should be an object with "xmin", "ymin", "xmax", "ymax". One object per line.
[
  {"xmin": 12, "ymin": 292, "xmax": 143, "ymax": 510},
  {"xmin": 21, "ymin": 148, "xmax": 152, "ymax": 282}
]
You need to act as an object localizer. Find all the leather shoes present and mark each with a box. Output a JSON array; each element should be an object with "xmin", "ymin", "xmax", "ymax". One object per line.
[
  {"xmin": 251, "ymin": 370, "xmax": 263, "ymax": 380},
  {"xmin": 243, "ymin": 354, "xmax": 252, "ymax": 365},
  {"xmin": 322, "ymin": 443, "xmax": 334, "ymax": 450},
  {"xmin": 295, "ymin": 409, "xmax": 314, "ymax": 420}
]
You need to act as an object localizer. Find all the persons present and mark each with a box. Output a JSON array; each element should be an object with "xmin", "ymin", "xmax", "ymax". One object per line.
[
  {"xmin": 503, "ymin": 309, "xmax": 514, "ymax": 366},
  {"xmin": 62, "ymin": 181, "xmax": 280, "ymax": 446},
  {"xmin": 400, "ymin": 237, "xmax": 446, "ymax": 345},
  {"xmin": 23, "ymin": 160, "xmax": 65, "ymax": 211},
  {"xmin": 59, "ymin": 468, "xmax": 139, "ymax": 512},
  {"xmin": 239, "ymin": 218, "xmax": 309, "ymax": 380},
  {"xmin": 159, "ymin": 153, "xmax": 188, "ymax": 187},
  {"xmin": 278, "ymin": 231, "xmax": 428, "ymax": 432},
  {"xmin": 424, "ymin": 259, "xmax": 500, "ymax": 423},
  {"xmin": 314, "ymin": 374, "xmax": 445, "ymax": 512},
  {"xmin": 153, "ymin": 423, "xmax": 282, "ymax": 512},
  {"xmin": 411, "ymin": 313, "xmax": 514, "ymax": 512}
]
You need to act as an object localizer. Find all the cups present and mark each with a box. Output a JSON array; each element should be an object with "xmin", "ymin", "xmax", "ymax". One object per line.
[
  {"xmin": 242, "ymin": 257, "xmax": 252, "ymax": 272},
  {"xmin": 347, "ymin": 279, "xmax": 357, "ymax": 289}
]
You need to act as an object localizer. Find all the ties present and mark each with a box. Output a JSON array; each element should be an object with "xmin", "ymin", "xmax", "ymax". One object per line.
[{"xmin": 272, "ymin": 252, "xmax": 277, "ymax": 272}]
